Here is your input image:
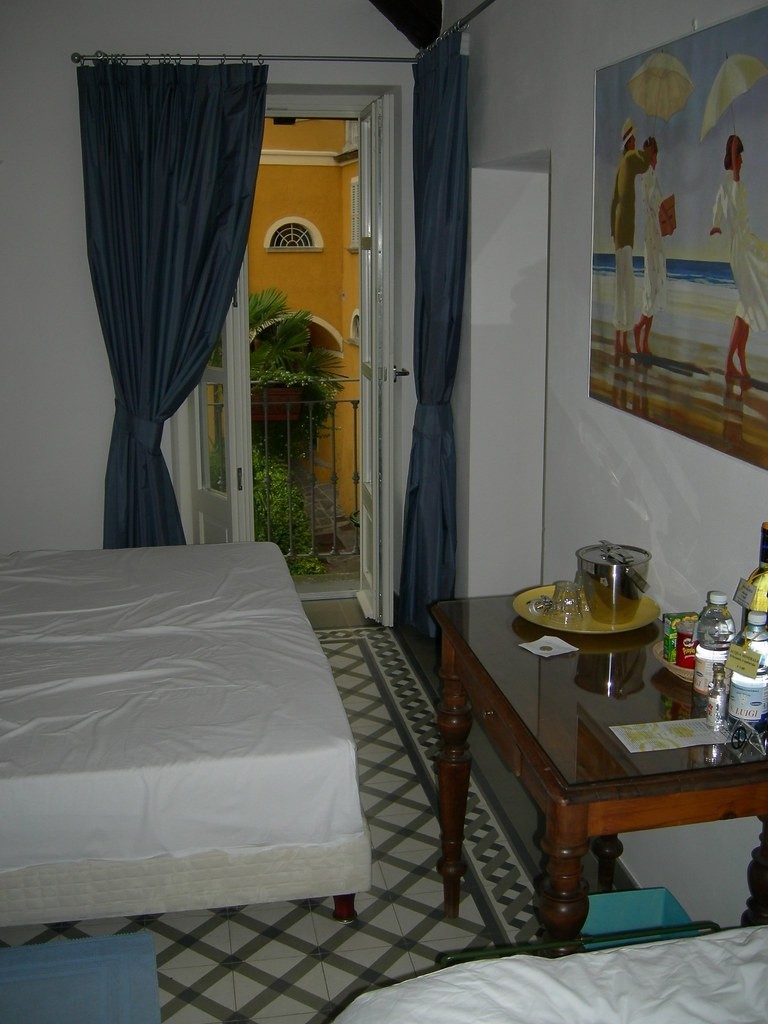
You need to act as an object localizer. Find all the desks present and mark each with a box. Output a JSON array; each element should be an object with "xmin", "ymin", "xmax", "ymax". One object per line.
[{"xmin": 430, "ymin": 594, "xmax": 767, "ymax": 945}]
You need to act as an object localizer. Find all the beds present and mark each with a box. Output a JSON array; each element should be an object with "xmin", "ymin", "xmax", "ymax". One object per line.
[{"xmin": 0, "ymin": 541, "xmax": 373, "ymax": 927}]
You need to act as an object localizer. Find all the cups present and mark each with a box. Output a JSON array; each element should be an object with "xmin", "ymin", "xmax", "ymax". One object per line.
[{"xmin": 548, "ymin": 581, "xmax": 584, "ymax": 624}]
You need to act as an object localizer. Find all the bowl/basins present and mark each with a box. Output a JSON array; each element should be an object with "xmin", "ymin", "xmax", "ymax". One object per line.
[{"xmin": 653, "ymin": 640, "xmax": 694, "ymax": 682}]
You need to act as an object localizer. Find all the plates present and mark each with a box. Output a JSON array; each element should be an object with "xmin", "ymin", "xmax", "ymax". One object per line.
[{"xmin": 511, "ymin": 585, "xmax": 660, "ymax": 634}]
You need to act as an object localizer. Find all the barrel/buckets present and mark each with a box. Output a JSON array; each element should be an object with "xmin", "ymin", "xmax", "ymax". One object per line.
[{"xmin": 577, "ymin": 543, "xmax": 652, "ymax": 624}]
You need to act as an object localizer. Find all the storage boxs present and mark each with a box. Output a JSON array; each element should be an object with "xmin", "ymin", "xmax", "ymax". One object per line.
[{"xmin": 577, "ymin": 887, "xmax": 700, "ymax": 952}]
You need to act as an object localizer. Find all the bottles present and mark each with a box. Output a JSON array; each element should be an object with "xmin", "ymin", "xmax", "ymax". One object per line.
[
  {"xmin": 691, "ymin": 592, "xmax": 736, "ymax": 711},
  {"xmin": 726, "ymin": 611, "xmax": 768, "ymax": 737},
  {"xmin": 707, "ymin": 663, "xmax": 726, "ymax": 694},
  {"xmin": 706, "ymin": 673, "xmax": 726, "ymax": 733}
]
